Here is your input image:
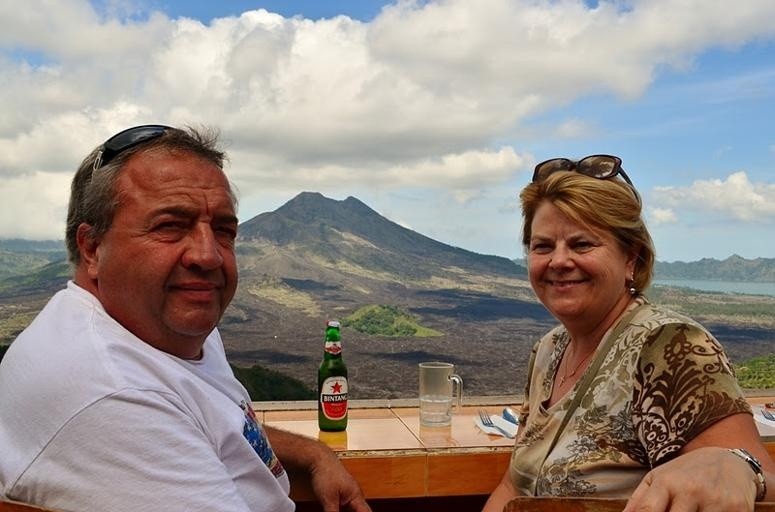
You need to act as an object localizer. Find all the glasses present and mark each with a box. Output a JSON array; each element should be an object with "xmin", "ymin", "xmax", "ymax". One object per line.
[
  {"xmin": 90, "ymin": 125, "xmax": 182, "ymax": 179},
  {"xmin": 533, "ymin": 155, "xmax": 633, "ymax": 186}
]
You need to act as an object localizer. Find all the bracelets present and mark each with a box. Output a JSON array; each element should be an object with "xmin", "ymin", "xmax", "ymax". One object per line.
[{"xmin": 728, "ymin": 445, "xmax": 768, "ymax": 502}]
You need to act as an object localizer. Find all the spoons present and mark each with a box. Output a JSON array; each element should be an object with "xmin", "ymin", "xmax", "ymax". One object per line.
[{"xmin": 503, "ymin": 407, "xmax": 519, "ymax": 424}]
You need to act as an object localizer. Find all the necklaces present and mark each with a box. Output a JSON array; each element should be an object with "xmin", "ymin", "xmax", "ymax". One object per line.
[{"xmin": 559, "ymin": 338, "xmax": 598, "ymax": 389}]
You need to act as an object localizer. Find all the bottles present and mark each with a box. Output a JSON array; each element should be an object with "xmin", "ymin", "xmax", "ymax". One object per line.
[{"xmin": 317, "ymin": 319, "xmax": 350, "ymax": 433}]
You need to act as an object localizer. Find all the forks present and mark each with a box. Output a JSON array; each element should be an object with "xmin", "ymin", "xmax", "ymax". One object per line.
[
  {"xmin": 761, "ymin": 409, "xmax": 775, "ymax": 421},
  {"xmin": 479, "ymin": 408, "xmax": 515, "ymax": 439}
]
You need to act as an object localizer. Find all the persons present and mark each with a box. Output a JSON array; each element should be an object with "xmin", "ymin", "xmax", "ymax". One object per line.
[
  {"xmin": 481, "ymin": 154, "xmax": 775, "ymax": 512},
  {"xmin": 0, "ymin": 122, "xmax": 373, "ymax": 512}
]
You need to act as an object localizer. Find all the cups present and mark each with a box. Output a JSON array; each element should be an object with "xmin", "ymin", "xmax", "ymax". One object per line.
[{"xmin": 417, "ymin": 361, "xmax": 464, "ymax": 429}]
